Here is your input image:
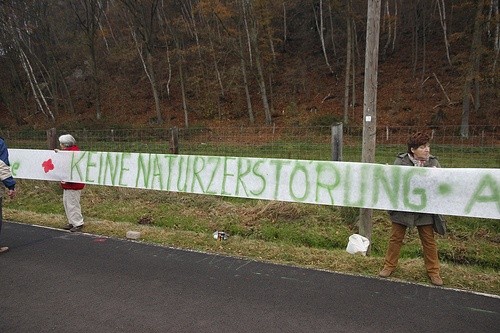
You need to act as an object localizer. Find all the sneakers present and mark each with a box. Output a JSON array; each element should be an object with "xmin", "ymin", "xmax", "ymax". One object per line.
[
  {"xmin": 428, "ymin": 273, "xmax": 443, "ymax": 285},
  {"xmin": 379, "ymin": 268, "xmax": 392, "ymax": 277}
]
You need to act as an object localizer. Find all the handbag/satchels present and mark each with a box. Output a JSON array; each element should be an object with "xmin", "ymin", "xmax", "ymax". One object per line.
[{"xmin": 433, "ymin": 214, "xmax": 445, "ymax": 235}]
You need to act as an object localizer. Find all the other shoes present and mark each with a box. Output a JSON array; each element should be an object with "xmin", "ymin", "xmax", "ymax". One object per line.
[
  {"xmin": 70, "ymin": 225, "xmax": 82, "ymax": 232},
  {"xmin": 0, "ymin": 247, "xmax": 8, "ymax": 253},
  {"xmin": 65, "ymin": 224, "xmax": 73, "ymax": 230}
]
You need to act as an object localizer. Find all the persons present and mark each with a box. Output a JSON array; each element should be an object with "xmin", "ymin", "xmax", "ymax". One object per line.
[
  {"xmin": 57, "ymin": 133, "xmax": 85, "ymax": 232},
  {"xmin": 378, "ymin": 132, "xmax": 447, "ymax": 286},
  {"xmin": 0, "ymin": 137, "xmax": 17, "ymax": 252}
]
